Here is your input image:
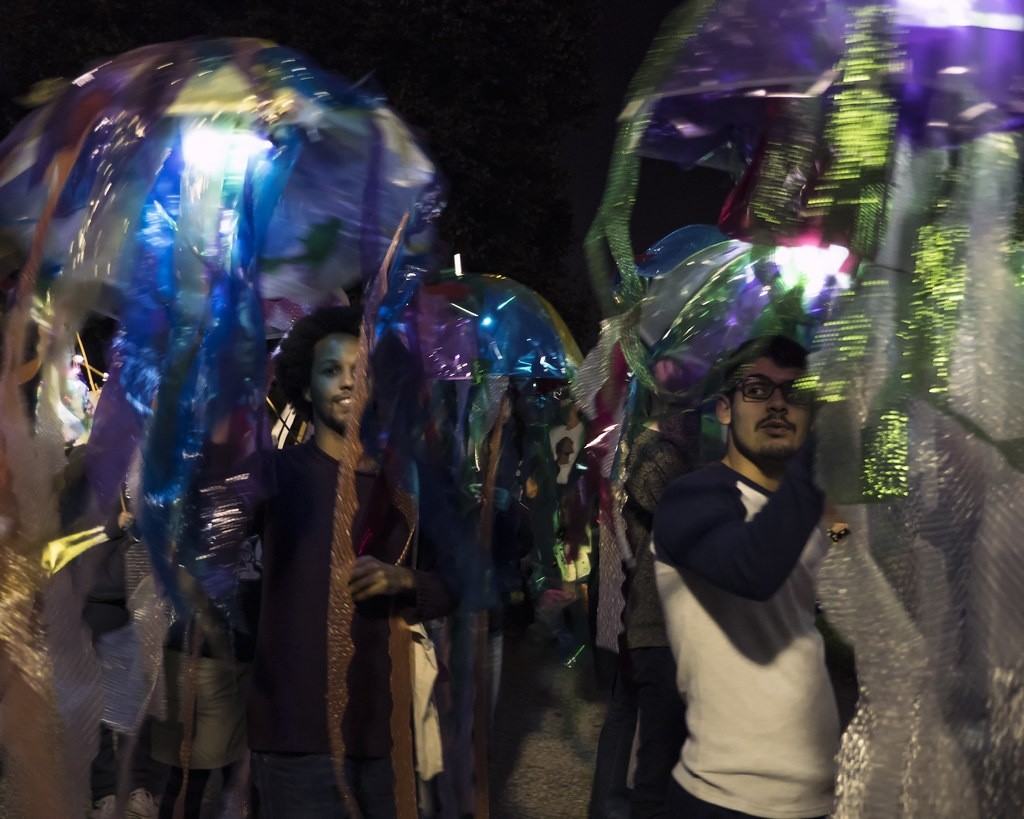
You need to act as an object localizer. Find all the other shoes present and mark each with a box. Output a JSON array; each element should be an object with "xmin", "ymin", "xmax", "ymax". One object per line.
[
  {"xmin": 122, "ymin": 786, "xmax": 161, "ymax": 819},
  {"xmin": 97, "ymin": 795, "xmax": 116, "ymax": 818}
]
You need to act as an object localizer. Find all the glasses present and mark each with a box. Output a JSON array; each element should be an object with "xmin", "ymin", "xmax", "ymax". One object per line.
[{"xmin": 736, "ymin": 379, "xmax": 810, "ymax": 406}]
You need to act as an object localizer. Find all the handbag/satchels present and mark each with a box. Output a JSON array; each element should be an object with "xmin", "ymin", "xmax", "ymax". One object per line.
[{"xmin": 405, "ymin": 624, "xmax": 445, "ymax": 782}]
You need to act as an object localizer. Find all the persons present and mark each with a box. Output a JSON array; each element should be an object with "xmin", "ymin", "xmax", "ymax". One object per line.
[
  {"xmin": 548, "ymin": 391, "xmax": 593, "ymax": 627},
  {"xmin": 61, "ymin": 308, "xmax": 522, "ymax": 819},
  {"xmin": 586, "ymin": 332, "xmax": 847, "ymax": 819}
]
